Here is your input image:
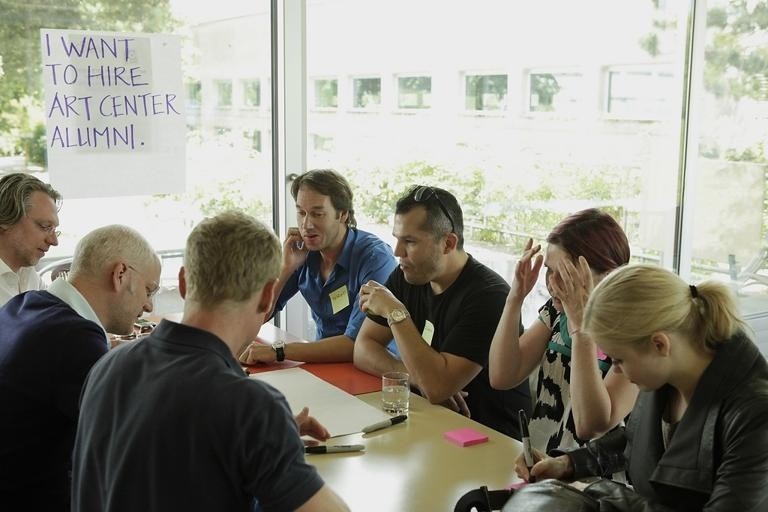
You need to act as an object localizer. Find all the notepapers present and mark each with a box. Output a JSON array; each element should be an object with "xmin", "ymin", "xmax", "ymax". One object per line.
[{"xmin": 444, "ymin": 427, "xmax": 489, "ymax": 448}]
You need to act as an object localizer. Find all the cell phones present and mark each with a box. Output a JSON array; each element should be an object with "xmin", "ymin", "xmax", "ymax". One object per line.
[{"xmin": 293, "ymin": 233, "xmax": 305, "ymax": 251}]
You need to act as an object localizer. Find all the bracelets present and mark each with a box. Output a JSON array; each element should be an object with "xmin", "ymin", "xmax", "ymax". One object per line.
[{"xmin": 567, "ymin": 328, "xmax": 581, "ymax": 340}]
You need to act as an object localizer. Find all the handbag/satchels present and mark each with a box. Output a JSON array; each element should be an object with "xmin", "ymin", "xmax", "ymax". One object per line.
[{"xmin": 500, "ymin": 477, "xmax": 623, "ymax": 512}]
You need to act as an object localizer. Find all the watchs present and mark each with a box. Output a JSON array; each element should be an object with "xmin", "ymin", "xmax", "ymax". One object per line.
[
  {"xmin": 383, "ymin": 307, "xmax": 411, "ymax": 327},
  {"xmin": 271, "ymin": 339, "xmax": 287, "ymax": 361}
]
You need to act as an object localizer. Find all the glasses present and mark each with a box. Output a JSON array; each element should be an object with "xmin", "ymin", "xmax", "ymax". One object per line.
[
  {"xmin": 404, "ymin": 184, "xmax": 457, "ymax": 234},
  {"xmin": 24, "ymin": 212, "xmax": 61, "ymax": 238},
  {"xmin": 126, "ymin": 262, "xmax": 161, "ymax": 297}
]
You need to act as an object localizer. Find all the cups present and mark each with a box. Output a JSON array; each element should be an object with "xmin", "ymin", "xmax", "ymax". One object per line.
[{"xmin": 380, "ymin": 372, "xmax": 411, "ymax": 413}]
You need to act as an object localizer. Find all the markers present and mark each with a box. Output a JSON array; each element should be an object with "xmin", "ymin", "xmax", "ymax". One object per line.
[
  {"xmin": 361, "ymin": 415, "xmax": 407, "ymax": 433},
  {"xmin": 304, "ymin": 444, "xmax": 365, "ymax": 453},
  {"xmin": 519, "ymin": 409, "xmax": 536, "ymax": 483}
]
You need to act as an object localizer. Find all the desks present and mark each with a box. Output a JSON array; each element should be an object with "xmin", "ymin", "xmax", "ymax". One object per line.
[{"xmin": 108, "ymin": 312, "xmax": 591, "ymax": 512}]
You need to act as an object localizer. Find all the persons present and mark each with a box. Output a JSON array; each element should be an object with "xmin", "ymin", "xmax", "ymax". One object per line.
[
  {"xmin": 514, "ymin": 263, "xmax": 768, "ymax": 512},
  {"xmin": 489, "ymin": 209, "xmax": 641, "ymax": 456},
  {"xmin": 353, "ymin": 186, "xmax": 532, "ymax": 439},
  {"xmin": 238, "ymin": 169, "xmax": 402, "ymax": 367},
  {"xmin": 0, "ymin": 224, "xmax": 161, "ymax": 512},
  {"xmin": 70, "ymin": 209, "xmax": 351, "ymax": 512},
  {"xmin": 0, "ymin": 173, "xmax": 63, "ymax": 309}
]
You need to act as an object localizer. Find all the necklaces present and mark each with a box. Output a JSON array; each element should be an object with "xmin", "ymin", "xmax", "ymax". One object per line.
[{"xmin": 665, "ymin": 400, "xmax": 674, "ymax": 423}]
